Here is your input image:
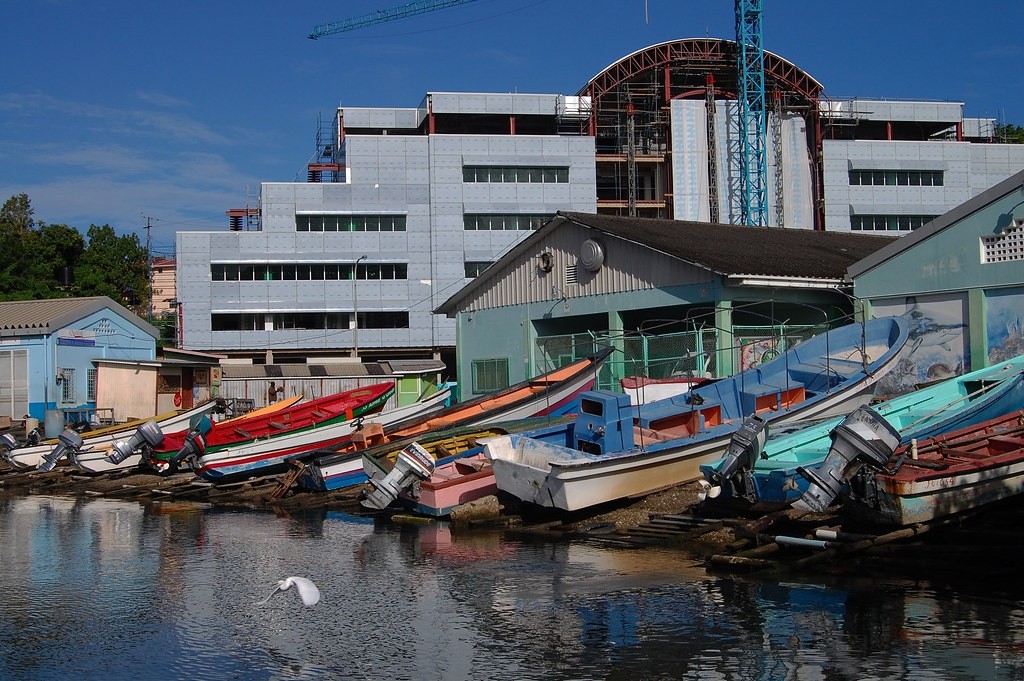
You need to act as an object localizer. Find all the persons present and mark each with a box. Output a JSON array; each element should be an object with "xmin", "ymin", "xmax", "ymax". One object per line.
[{"xmin": 268, "ymin": 382, "xmax": 280, "ymax": 405}]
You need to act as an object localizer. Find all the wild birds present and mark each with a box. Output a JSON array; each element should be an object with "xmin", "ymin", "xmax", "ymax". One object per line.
[{"xmin": 255, "ymin": 577, "xmax": 320, "ymax": 607}]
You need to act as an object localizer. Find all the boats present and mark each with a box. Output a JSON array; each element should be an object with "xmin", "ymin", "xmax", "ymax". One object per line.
[
  {"xmin": 0, "ymin": 400, "xmax": 224, "ymax": 470},
  {"xmin": 470, "ymin": 315, "xmax": 910, "ymax": 514},
  {"xmin": 262, "ymin": 344, "xmax": 617, "ymax": 505},
  {"xmin": 356, "ymin": 412, "xmax": 579, "ymax": 517},
  {"xmin": 37, "ymin": 394, "xmax": 303, "ymax": 475},
  {"xmin": 790, "ymin": 405, "xmax": 1024, "ymax": 528},
  {"xmin": 165, "ymin": 378, "xmax": 456, "ymax": 487},
  {"xmin": 698, "ymin": 354, "xmax": 1023, "ymax": 520},
  {"xmin": 103, "ymin": 382, "xmax": 397, "ymax": 477}
]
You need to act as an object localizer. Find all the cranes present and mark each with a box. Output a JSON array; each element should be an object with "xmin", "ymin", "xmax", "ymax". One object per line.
[{"xmin": 311, "ymin": 0, "xmax": 769, "ymax": 226}]
